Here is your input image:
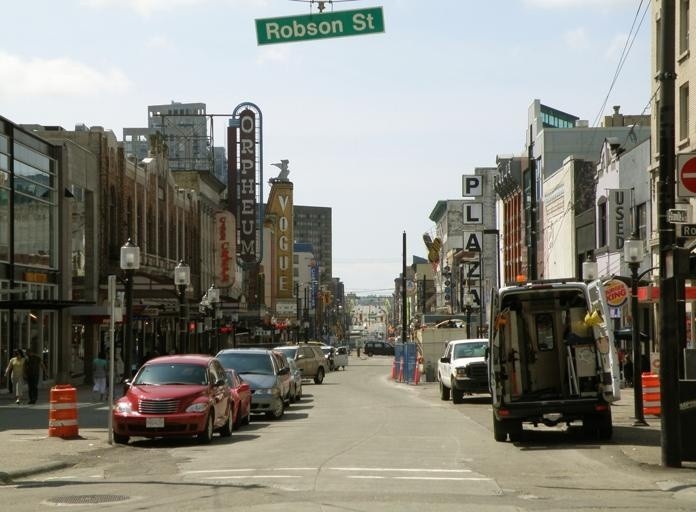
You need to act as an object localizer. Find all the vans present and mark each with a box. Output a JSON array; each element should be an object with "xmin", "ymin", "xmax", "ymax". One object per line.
[
  {"xmin": 285, "ymin": 340, "xmax": 326, "ymax": 346},
  {"xmin": 489, "ymin": 279, "xmax": 623, "ymax": 444}
]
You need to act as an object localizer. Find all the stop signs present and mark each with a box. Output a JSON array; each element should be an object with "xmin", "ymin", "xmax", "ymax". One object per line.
[{"xmin": 676, "ymin": 152, "xmax": 696, "ymax": 198}]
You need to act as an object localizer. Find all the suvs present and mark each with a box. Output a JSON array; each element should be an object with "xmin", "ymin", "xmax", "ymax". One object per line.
[
  {"xmin": 270, "ymin": 345, "xmax": 330, "ymax": 383},
  {"xmin": 437, "ymin": 338, "xmax": 490, "ymax": 404},
  {"xmin": 364, "ymin": 341, "xmax": 395, "ymax": 356}
]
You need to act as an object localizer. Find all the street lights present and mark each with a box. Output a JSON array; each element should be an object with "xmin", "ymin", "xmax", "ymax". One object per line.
[
  {"xmin": 231, "ymin": 311, "xmax": 240, "ymax": 348},
  {"xmin": 482, "ymin": 227, "xmax": 501, "ymax": 290},
  {"xmin": 205, "ymin": 284, "xmax": 220, "ymax": 359},
  {"xmin": 293, "ymin": 282, "xmax": 340, "ymax": 338},
  {"xmin": 117, "ymin": 237, "xmax": 143, "ymax": 398},
  {"xmin": 172, "ymin": 258, "xmax": 191, "ymax": 354},
  {"xmin": 214, "ymin": 307, "xmax": 224, "ymax": 351},
  {"xmin": 269, "ymin": 316, "xmax": 310, "ymax": 346},
  {"xmin": 464, "ymin": 291, "xmax": 475, "ymax": 340},
  {"xmin": 613, "ymin": 231, "xmax": 654, "ymax": 425}
]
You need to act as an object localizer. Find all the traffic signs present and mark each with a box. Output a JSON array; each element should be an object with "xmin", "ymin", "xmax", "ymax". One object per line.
[
  {"xmin": 255, "ymin": 5, "xmax": 385, "ymax": 47},
  {"xmin": 681, "ymin": 223, "xmax": 696, "ymax": 238}
]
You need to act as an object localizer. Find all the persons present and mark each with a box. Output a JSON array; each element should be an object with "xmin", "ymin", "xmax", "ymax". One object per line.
[
  {"xmin": 23, "ymin": 349, "xmax": 48, "ymax": 404},
  {"xmin": 4, "ymin": 349, "xmax": 26, "ymax": 403},
  {"xmin": 105, "ymin": 352, "xmax": 121, "ymax": 401},
  {"xmin": 92, "ymin": 350, "xmax": 107, "ymax": 402},
  {"xmin": 142, "ymin": 344, "xmax": 176, "ymax": 363},
  {"xmin": 641, "ymin": 354, "xmax": 649, "ymax": 373},
  {"xmin": 623, "ymin": 354, "xmax": 633, "ymax": 388}
]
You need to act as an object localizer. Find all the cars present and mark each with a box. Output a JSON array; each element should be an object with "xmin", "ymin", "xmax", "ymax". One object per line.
[
  {"xmin": 347, "ymin": 323, "xmax": 383, "ymax": 345},
  {"xmin": 321, "ymin": 346, "xmax": 348, "ymax": 370},
  {"xmin": 112, "ymin": 355, "xmax": 235, "ymax": 443},
  {"xmin": 211, "ymin": 348, "xmax": 302, "ymax": 428}
]
